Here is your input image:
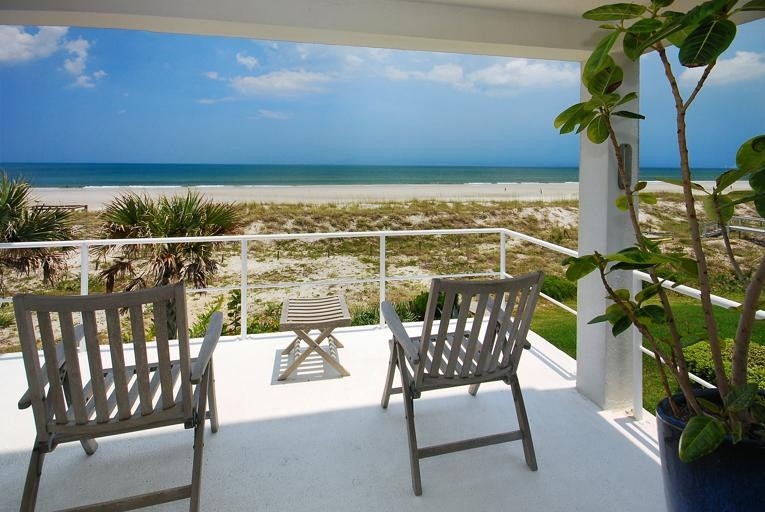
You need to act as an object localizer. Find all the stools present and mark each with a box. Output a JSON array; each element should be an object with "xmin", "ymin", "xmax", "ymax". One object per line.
[{"xmin": 277, "ymin": 292, "xmax": 355, "ymax": 380}]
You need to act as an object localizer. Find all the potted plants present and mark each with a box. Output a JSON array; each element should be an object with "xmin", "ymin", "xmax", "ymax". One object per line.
[{"xmin": 554, "ymin": 2, "xmax": 765, "ymax": 512}]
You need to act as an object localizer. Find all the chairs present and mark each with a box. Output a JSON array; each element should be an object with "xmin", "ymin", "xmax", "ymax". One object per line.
[
  {"xmin": 378, "ymin": 265, "xmax": 547, "ymax": 497},
  {"xmin": 11, "ymin": 277, "xmax": 225, "ymax": 512}
]
